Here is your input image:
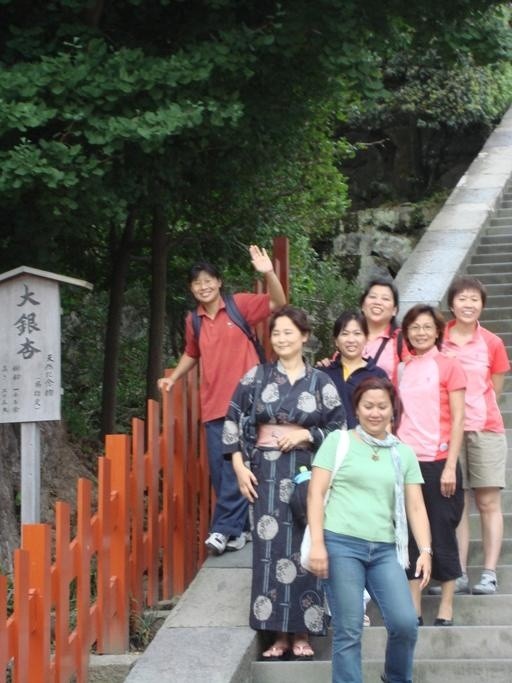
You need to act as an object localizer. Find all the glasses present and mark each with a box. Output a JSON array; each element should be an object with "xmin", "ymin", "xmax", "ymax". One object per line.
[{"xmin": 407, "ymin": 322, "xmax": 438, "ymax": 333}]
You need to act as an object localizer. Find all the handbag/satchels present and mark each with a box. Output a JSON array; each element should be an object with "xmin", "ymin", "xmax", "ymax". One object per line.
[
  {"xmin": 300, "ymin": 490, "xmax": 331, "ymax": 571},
  {"xmin": 240, "ymin": 416, "xmax": 259, "ymax": 461}
]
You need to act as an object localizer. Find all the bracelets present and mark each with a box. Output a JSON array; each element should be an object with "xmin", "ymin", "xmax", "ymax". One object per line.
[
  {"xmin": 419, "ymin": 547, "xmax": 434, "ymax": 556},
  {"xmin": 370, "ymin": 444, "xmax": 381, "ymax": 460}
]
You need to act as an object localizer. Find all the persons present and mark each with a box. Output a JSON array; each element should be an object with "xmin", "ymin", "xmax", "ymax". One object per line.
[
  {"xmin": 391, "ymin": 303, "xmax": 468, "ymax": 626},
  {"xmin": 221, "ymin": 302, "xmax": 348, "ymax": 662},
  {"xmin": 154, "ymin": 240, "xmax": 289, "ymax": 558},
  {"xmin": 312, "ymin": 307, "xmax": 393, "ymax": 629},
  {"xmin": 306, "ymin": 377, "xmax": 436, "ymax": 682},
  {"xmin": 312, "ymin": 275, "xmax": 419, "ymax": 396},
  {"xmin": 429, "ymin": 274, "xmax": 511, "ymax": 597}
]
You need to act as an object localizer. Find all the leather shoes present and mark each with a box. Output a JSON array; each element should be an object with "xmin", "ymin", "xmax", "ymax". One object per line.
[
  {"xmin": 416, "ymin": 615, "xmax": 423, "ymax": 625},
  {"xmin": 434, "ymin": 617, "xmax": 454, "ymax": 626}
]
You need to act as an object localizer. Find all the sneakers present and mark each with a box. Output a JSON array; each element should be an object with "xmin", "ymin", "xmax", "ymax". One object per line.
[
  {"xmin": 471, "ymin": 573, "xmax": 499, "ymax": 594},
  {"xmin": 227, "ymin": 530, "xmax": 252, "ymax": 550},
  {"xmin": 428, "ymin": 576, "xmax": 471, "ymax": 596},
  {"xmin": 204, "ymin": 532, "xmax": 230, "ymax": 556}
]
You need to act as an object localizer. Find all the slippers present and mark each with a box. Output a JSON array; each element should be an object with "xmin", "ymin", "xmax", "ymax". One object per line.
[
  {"xmin": 290, "ymin": 644, "xmax": 315, "ymax": 661},
  {"xmin": 262, "ymin": 645, "xmax": 292, "ymax": 661}
]
